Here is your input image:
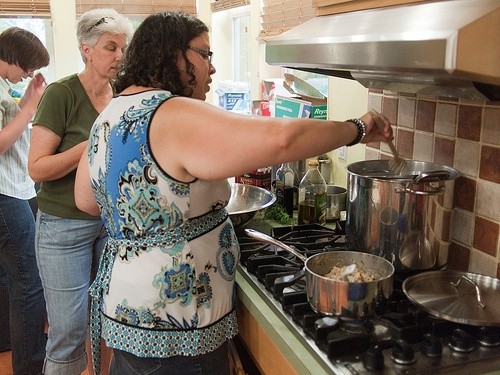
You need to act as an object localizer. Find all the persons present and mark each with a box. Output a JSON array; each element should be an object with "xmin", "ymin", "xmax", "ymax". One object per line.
[
  {"xmin": 74, "ymin": 12, "xmax": 396, "ymax": 375},
  {"xmin": 27, "ymin": 7, "xmax": 134, "ymax": 375},
  {"xmin": 0, "ymin": 27, "xmax": 50, "ymax": 375}
]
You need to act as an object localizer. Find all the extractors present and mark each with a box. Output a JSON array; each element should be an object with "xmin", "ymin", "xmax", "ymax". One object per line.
[{"xmin": 264, "ymin": 1, "xmax": 500, "ymax": 104}]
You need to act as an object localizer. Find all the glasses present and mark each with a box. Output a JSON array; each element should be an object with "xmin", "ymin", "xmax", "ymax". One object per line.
[
  {"xmin": 87, "ymin": 16, "xmax": 115, "ymax": 32},
  {"xmin": 189, "ymin": 46, "xmax": 213, "ymax": 64}
]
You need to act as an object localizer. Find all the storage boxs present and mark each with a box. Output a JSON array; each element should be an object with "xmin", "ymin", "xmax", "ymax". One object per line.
[{"xmin": 214, "ymin": 73, "xmax": 327, "ymax": 119}]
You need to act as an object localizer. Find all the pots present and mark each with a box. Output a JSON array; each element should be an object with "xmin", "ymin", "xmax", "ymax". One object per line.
[
  {"xmin": 246, "ymin": 227, "xmax": 394, "ymax": 317},
  {"xmin": 345, "ymin": 159, "xmax": 462, "ymax": 283}
]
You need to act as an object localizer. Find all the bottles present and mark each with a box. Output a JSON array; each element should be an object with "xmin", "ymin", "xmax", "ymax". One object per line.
[
  {"xmin": 277, "ymin": 161, "xmax": 294, "ymax": 218},
  {"xmin": 297, "ymin": 159, "xmax": 327, "ymax": 226}
]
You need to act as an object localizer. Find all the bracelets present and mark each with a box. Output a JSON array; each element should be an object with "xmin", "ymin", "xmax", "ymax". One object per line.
[{"xmin": 345, "ymin": 119, "xmax": 366, "ymax": 146}]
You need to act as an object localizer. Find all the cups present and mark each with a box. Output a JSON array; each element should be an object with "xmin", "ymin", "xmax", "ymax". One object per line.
[{"xmin": 306, "ymin": 184, "xmax": 348, "ymax": 219}]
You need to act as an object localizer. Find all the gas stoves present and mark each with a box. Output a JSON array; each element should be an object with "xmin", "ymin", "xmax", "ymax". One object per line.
[{"xmin": 235, "ymin": 223, "xmax": 500, "ymax": 375}]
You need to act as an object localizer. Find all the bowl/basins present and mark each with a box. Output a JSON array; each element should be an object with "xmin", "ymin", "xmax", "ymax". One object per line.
[{"xmin": 222, "ymin": 183, "xmax": 278, "ymax": 230}]
